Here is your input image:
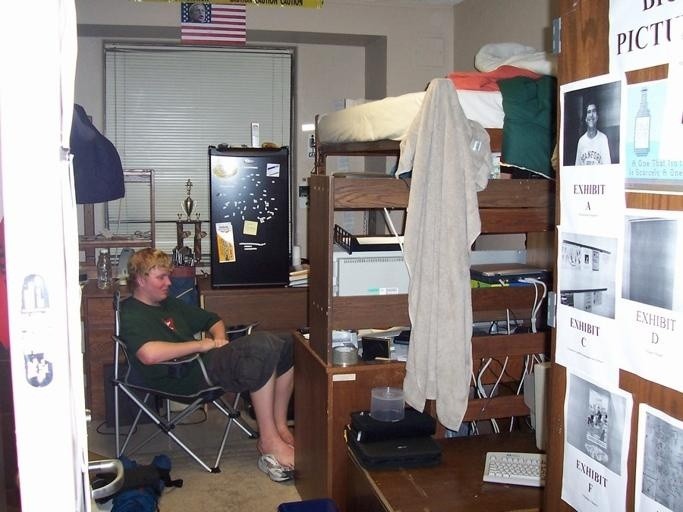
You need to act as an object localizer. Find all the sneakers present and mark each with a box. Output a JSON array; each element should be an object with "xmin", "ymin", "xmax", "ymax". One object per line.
[{"xmin": 256, "ymin": 454, "xmax": 294, "ymax": 482}]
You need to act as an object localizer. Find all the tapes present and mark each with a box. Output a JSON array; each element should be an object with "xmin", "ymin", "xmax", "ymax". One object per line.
[{"xmin": 333, "ymin": 346, "xmax": 357, "ymax": 366}]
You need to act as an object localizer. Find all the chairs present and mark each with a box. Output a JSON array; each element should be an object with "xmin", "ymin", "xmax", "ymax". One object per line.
[{"xmin": 109, "ymin": 276, "xmax": 260, "ymax": 474}]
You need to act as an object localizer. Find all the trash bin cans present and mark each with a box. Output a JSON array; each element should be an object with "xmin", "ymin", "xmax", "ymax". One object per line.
[
  {"xmin": 88, "ymin": 459, "xmax": 124, "ymax": 512},
  {"xmin": 277, "ymin": 498, "xmax": 337, "ymax": 512}
]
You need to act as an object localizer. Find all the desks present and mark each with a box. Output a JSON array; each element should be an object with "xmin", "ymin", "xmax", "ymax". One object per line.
[{"xmin": 81, "ymin": 281, "xmax": 308, "ymax": 422}]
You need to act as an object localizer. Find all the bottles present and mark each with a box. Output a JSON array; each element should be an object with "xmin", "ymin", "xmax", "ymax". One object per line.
[
  {"xmin": 633, "ymin": 88, "xmax": 651, "ymax": 158},
  {"xmin": 97, "ymin": 249, "xmax": 113, "ymax": 290}
]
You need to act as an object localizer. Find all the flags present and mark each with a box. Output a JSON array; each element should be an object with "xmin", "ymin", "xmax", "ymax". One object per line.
[{"xmin": 181, "ymin": 1, "xmax": 248, "ymax": 46}]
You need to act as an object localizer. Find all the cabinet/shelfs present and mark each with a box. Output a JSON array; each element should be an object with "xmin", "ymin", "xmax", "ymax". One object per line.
[{"xmin": 292, "ymin": 174, "xmax": 554, "ymax": 510}]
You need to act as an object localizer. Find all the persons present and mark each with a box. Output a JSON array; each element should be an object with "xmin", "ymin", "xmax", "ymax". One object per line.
[
  {"xmin": 575, "ymin": 102, "xmax": 612, "ymax": 167},
  {"xmin": 188, "ymin": 2, "xmax": 206, "ymax": 23},
  {"xmin": 116, "ymin": 248, "xmax": 296, "ymax": 469}
]
